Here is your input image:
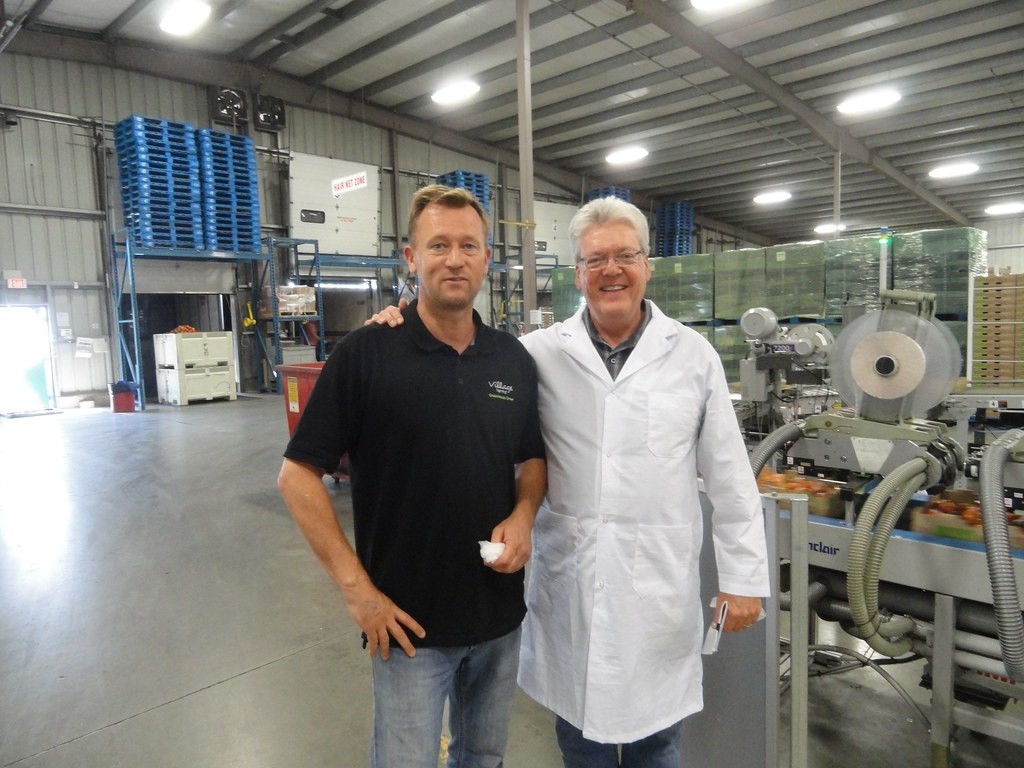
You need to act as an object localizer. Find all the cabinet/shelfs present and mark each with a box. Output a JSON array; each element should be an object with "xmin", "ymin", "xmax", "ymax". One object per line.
[
  {"xmin": 154, "ymin": 331, "xmax": 237, "ymax": 406},
  {"xmin": 250, "ymin": 237, "xmax": 326, "ymax": 395}
]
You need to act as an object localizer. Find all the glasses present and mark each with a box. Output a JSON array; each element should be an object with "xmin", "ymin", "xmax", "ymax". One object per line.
[{"xmin": 578, "ymin": 250, "xmax": 643, "ymax": 272}]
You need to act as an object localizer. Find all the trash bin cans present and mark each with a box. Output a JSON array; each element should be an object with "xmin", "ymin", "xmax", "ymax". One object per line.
[{"xmin": 107, "ymin": 382, "xmax": 135, "ymax": 413}]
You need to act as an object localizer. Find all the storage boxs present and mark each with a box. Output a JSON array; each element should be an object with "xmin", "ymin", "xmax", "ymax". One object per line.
[
  {"xmin": 759, "ymin": 471, "xmax": 1024, "ymax": 551},
  {"xmin": 276, "ymin": 362, "xmax": 351, "ymax": 482},
  {"xmin": 972, "ymin": 274, "xmax": 1024, "ymax": 388},
  {"xmin": 270, "ymin": 344, "xmax": 316, "ymax": 381},
  {"xmin": 263, "ymin": 285, "xmax": 316, "ymax": 313}
]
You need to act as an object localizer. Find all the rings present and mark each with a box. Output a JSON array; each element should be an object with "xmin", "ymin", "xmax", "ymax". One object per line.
[{"xmin": 744, "ymin": 623, "xmax": 753, "ymax": 628}]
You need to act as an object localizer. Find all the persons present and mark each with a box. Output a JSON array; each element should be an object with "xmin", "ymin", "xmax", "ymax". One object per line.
[
  {"xmin": 277, "ymin": 185, "xmax": 547, "ymax": 768},
  {"xmin": 365, "ymin": 196, "xmax": 771, "ymax": 768}
]
